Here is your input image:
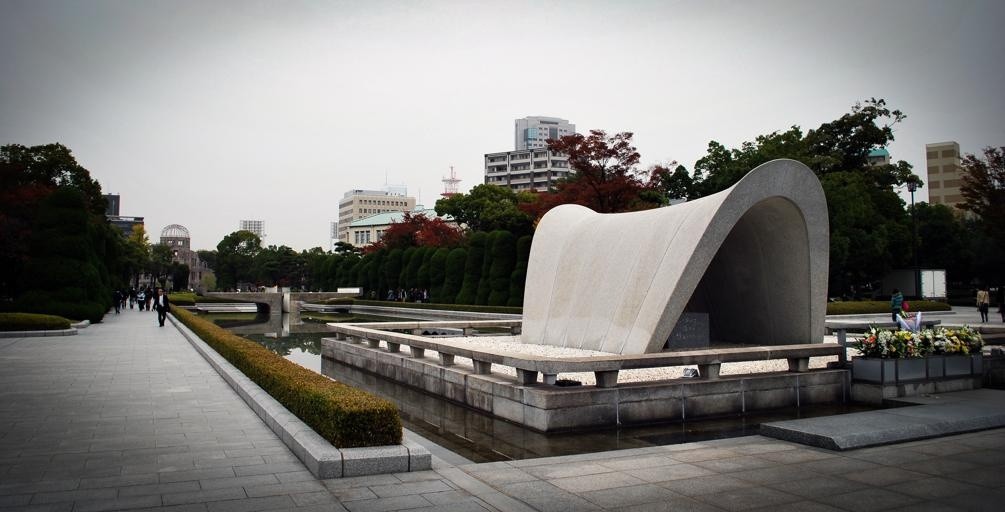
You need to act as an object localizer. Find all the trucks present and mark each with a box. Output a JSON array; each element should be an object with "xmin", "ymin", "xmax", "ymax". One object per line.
[{"xmin": 870, "ymin": 269, "xmax": 948, "ymax": 303}]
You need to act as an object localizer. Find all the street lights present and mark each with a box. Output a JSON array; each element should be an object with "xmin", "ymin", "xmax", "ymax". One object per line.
[{"xmin": 907, "ymin": 177, "xmax": 923, "ymax": 299}]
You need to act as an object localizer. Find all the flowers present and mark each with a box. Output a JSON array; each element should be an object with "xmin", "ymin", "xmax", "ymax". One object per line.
[{"xmin": 855, "ymin": 319, "xmax": 985, "ymax": 360}]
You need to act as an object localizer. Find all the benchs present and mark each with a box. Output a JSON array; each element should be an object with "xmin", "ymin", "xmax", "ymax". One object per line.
[{"xmin": 824, "ymin": 317, "xmax": 941, "ymax": 369}]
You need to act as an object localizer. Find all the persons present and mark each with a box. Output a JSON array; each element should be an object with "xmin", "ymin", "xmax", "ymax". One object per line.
[
  {"xmin": 111, "ymin": 284, "xmax": 154, "ymax": 314},
  {"xmin": 890, "ymin": 287, "xmax": 904, "ymax": 322},
  {"xmin": 154, "ymin": 283, "xmax": 329, "ymax": 295},
  {"xmin": 976, "ymin": 284, "xmax": 990, "ymax": 324},
  {"xmin": 366, "ymin": 286, "xmax": 432, "ymax": 303},
  {"xmin": 151, "ymin": 287, "xmax": 170, "ymax": 329}
]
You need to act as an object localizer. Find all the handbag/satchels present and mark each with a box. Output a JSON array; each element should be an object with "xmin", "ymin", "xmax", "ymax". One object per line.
[{"xmin": 901, "ymin": 299, "xmax": 909, "ymax": 312}]
